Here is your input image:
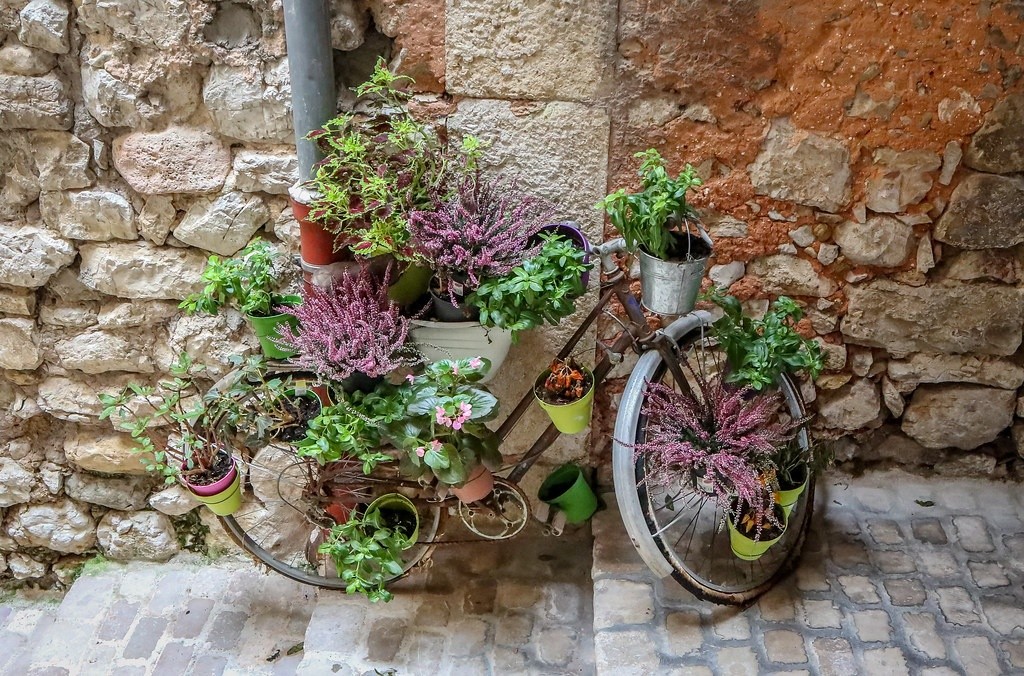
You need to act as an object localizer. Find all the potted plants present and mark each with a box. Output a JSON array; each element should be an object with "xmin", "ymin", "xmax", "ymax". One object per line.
[{"xmin": 97, "ymin": 52, "xmax": 830, "ymax": 604}]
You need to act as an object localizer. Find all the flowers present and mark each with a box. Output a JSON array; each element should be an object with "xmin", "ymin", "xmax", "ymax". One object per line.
[{"xmin": 385, "ymin": 352, "xmax": 504, "ymax": 485}]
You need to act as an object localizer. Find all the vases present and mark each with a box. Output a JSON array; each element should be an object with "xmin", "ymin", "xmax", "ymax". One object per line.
[{"xmin": 445, "ymin": 458, "xmax": 494, "ymax": 505}]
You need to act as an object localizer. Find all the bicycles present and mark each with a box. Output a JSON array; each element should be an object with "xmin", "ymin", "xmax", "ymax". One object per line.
[{"xmin": 188, "ymin": 222, "xmax": 817, "ymax": 607}]
